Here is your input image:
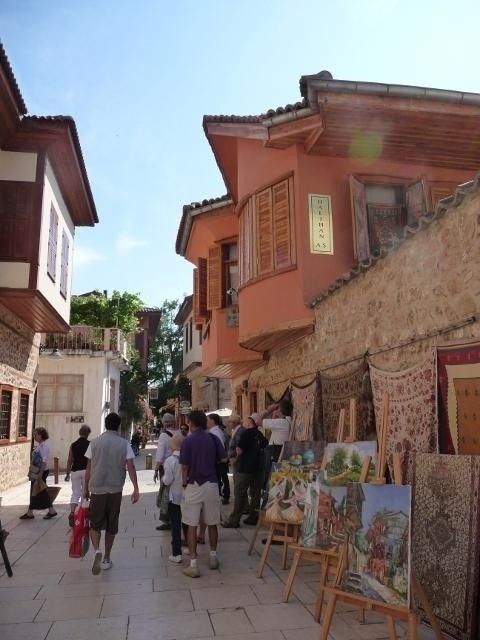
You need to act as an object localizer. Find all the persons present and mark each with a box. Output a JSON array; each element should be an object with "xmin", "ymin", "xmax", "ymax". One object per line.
[
  {"xmin": 153, "ymin": 399, "xmax": 293, "ymax": 578},
  {"xmin": 20, "ymin": 426, "xmax": 57, "ymax": 519},
  {"xmin": 63, "ymin": 425, "xmax": 90, "ymax": 526},
  {"xmin": 84, "ymin": 413, "xmax": 138, "ymax": 575}
]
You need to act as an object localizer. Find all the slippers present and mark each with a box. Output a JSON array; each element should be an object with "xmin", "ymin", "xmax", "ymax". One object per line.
[{"xmin": 18, "ymin": 512, "xmax": 58, "ymax": 519}]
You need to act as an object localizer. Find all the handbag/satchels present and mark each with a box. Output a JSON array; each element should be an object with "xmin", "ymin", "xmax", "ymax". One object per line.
[
  {"xmin": 26, "ymin": 466, "xmax": 41, "ymax": 481},
  {"xmin": 223, "ymin": 431, "xmax": 230, "ymax": 449},
  {"xmin": 68, "ymin": 497, "xmax": 89, "ymax": 560}
]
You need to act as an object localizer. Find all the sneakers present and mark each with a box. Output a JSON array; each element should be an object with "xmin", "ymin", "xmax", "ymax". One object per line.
[
  {"xmin": 221, "ymin": 498, "xmax": 229, "ymax": 504},
  {"xmin": 68, "ymin": 513, "xmax": 75, "ymax": 528},
  {"xmin": 243, "ymin": 517, "xmax": 258, "ymax": 525},
  {"xmin": 91, "ymin": 550, "xmax": 102, "ymax": 575},
  {"xmin": 100, "ymin": 560, "xmax": 112, "ymax": 570},
  {"xmin": 169, "ymin": 535, "xmax": 219, "ymax": 578},
  {"xmin": 220, "ymin": 519, "xmax": 240, "ymax": 528}
]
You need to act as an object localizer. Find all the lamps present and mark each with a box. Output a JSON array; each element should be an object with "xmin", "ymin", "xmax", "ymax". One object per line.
[
  {"xmin": 226, "ymin": 286, "xmax": 238, "ymax": 297},
  {"xmin": 39, "ymin": 339, "xmax": 63, "ymax": 359}
]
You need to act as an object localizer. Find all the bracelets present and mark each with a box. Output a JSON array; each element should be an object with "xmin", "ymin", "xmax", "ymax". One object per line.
[{"xmin": 66, "ymin": 473, "xmax": 70, "ymax": 475}]
